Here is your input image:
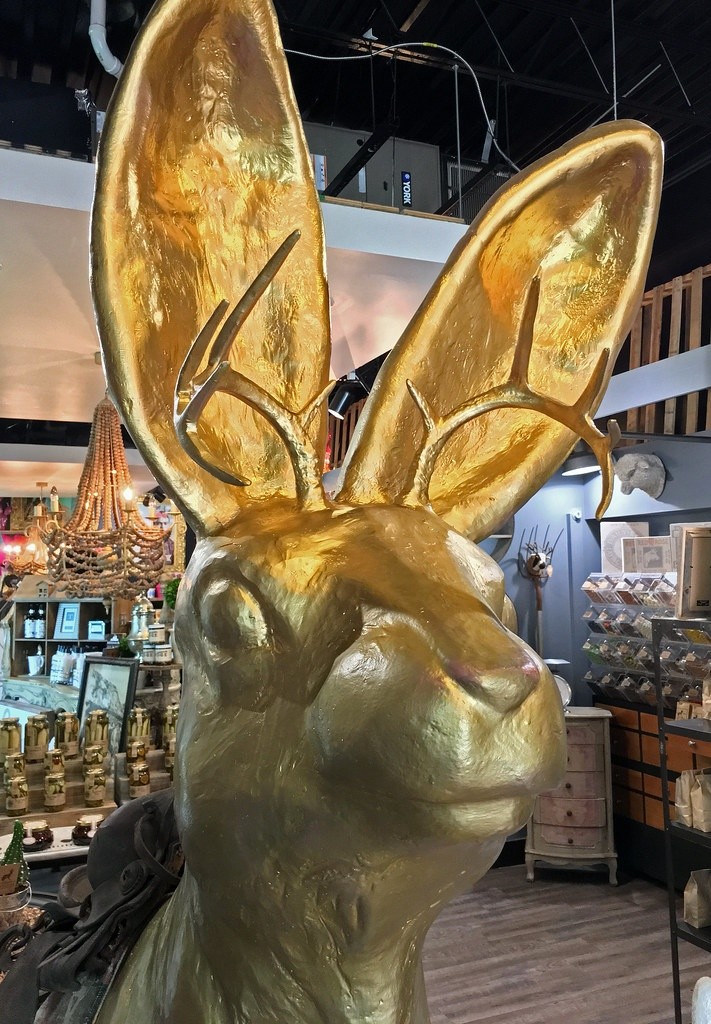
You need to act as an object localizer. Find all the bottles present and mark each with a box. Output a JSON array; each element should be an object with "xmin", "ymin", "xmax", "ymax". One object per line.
[
  {"xmin": 24, "ymin": 610, "xmax": 35, "ymax": 639},
  {"xmin": 22, "ymin": 820, "xmax": 53, "ymax": 853},
  {"xmin": 50, "ymin": 644, "xmax": 86, "ymax": 685},
  {"xmin": 72, "ymin": 813, "xmax": 105, "ymax": 846},
  {"xmin": 34, "ymin": 610, "xmax": 46, "ymax": 638}
]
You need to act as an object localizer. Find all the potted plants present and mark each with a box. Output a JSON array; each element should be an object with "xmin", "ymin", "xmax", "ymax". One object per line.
[{"xmin": 0, "ymin": 819, "xmax": 33, "ymax": 929}]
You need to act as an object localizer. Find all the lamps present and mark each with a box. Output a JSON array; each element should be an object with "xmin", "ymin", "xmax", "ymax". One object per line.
[
  {"xmin": 562, "ymin": 430, "xmax": 711, "ymax": 475},
  {"xmin": 0, "ymin": 355, "xmax": 172, "ymax": 602},
  {"xmin": 328, "ymin": 371, "xmax": 369, "ymax": 419}
]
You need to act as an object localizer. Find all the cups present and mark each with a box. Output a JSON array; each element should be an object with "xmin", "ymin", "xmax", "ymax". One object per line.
[{"xmin": 27, "ymin": 656, "xmax": 44, "ymax": 676}]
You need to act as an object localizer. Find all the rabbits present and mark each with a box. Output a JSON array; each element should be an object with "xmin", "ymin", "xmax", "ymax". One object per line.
[{"xmin": 11, "ymin": 0, "xmax": 673, "ymax": 1024}]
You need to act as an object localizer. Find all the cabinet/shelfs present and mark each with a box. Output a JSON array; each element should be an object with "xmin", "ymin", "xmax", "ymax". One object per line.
[
  {"xmin": 524, "ymin": 572, "xmax": 711, "ymax": 1024},
  {"xmin": 10, "ymin": 593, "xmax": 131, "ymax": 684}
]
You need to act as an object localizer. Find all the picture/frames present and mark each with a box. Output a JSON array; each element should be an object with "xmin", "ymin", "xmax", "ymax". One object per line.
[{"xmin": 77, "ymin": 655, "xmax": 139, "ymax": 753}]
